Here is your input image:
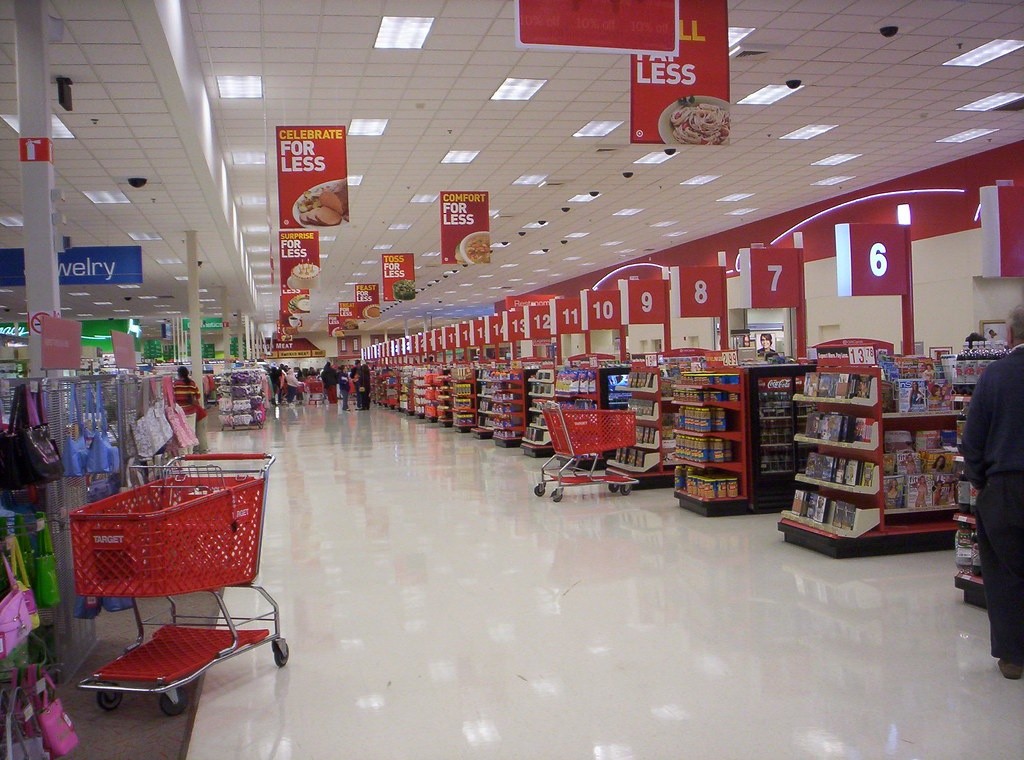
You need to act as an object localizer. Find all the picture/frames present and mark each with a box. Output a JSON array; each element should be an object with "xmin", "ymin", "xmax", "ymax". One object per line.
[
  {"xmin": 979, "ymin": 319, "xmax": 1007, "ymax": 340},
  {"xmin": 929, "ymin": 347, "xmax": 952, "ymax": 360}
]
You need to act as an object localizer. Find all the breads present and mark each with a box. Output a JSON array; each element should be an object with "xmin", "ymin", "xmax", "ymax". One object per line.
[{"xmin": 300, "ymin": 190, "xmax": 345, "ymax": 226}]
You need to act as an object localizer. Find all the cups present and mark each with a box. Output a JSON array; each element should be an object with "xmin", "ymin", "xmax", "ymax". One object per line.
[{"xmin": 288, "ymin": 317, "xmax": 301, "ymax": 327}]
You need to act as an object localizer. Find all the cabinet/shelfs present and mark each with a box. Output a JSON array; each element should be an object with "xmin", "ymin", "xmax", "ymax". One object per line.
[{"xmin": 367, "ymin": 339, "xmax": 986, "ymax": 610}]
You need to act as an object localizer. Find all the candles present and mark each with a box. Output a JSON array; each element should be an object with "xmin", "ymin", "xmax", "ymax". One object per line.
[{"xmin": 298, "ymin": 259, "xmax": 314, "ymax": 272}]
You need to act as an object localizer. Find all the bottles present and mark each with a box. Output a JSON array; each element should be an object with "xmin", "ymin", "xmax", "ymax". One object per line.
[
  {"xmin": 481, "ymin": 382, "xmax": 492, "ymax": 395},
  {"xmin": 425, "ymin": 403, "xmax": 436, "ymax": 416},
  {"xmin": 455, "ymin": 384, "xmax": 471, "ymax": 394},
  {"xmin": 425, "ymin": 387, "xmax": 440, "ymax": 400},
  {"xmin": 478, "ymin": 415, "xmax": 490, "ymax": 427},
  {"xmin": 678, "ymin": 406, "xmax": 726, "ymax": 431},
  {"xmin": 955, "ymin": 523, "xmax": 982, "ymax": 577},
  {"xmin": 952, "ymin": 341, "xmax": 1010, "ymax": 394},
  {"xmin": 729, "ymin": 393, "xmax": 737, "ymax": 400},
  {"xmin": 675, "ymin": 466, "xmax": 738, "ymax": 498},
  {"xmin": 681, "ymin": 375, "xmax": 719, "ymax": 385},
  {"xmin": 760, "ymin": 420, "xmax": 791, "ymax": 444},
  {"xmin": 958, "ymin": 471, "xmax": 982, "ymax": 515},
  {"xmin": 675, "ymin": 435, "xmax": 732, "ymax": 462},
  {"xmin": 759, "ymin": 392, "xmax": 791, "ymax": 417},
  {"xmin": 480, "ymin": 398, "xmax": 494, "ymax": 411},
  {"xmin": 760, "ymin": 446, "xmax": 790, "ymax": 471},
  {"xmin": 673, "ymin": 389, "xmax": 726, "ymax": 401},
  {"xmin": 956, "ymin": 397, "xmax": 970, "ymax": 456}
]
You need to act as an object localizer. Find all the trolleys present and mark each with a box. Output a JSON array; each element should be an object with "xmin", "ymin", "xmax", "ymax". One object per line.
[
  {"xmin": 534, "ymin": 401, "xmax": 641, "ymax": 504},
  {"xmin": 307, "ymin": 379, "xmax": 326, "ymax": 406},
  {"xmin": 68, "ymin": 449, "xmax": 289, "ymax": 717}
]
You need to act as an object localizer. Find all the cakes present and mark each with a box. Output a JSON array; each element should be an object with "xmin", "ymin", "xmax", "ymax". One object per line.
[{"xmin": 290, "ymin": 263, "xmax": 320, "ymax": 289}]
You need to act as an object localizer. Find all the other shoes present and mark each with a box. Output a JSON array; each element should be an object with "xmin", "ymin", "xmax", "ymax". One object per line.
[
  {"xmin": 998, "ymin": 660, "xmax": 1020, "ymax": 679},
  {"xmin": 345, "ymin": 407, "xmax": 350, "ymax": 411},
  {"xmin": 360, "ymin": 407, "xmax": 369, "ymax": 410}
]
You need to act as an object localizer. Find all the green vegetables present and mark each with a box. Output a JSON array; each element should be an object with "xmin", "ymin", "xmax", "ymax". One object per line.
[{"xmin": 676, "ymin": 96, "xmax": 695, "ymax": 107}]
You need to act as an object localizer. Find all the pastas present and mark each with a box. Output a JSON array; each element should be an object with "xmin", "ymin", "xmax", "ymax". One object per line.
[{"xmin": 671, "ymin": 104, "xmax": 731, "ymax": 146}]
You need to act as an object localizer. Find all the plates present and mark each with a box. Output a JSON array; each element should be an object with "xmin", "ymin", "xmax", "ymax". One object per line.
[
  {"xmin": 292, "ymin": 180, "xmax": 343, "ymax": 227},
  {"xmin": 332, "ymin": 304, "xmax": 380, "ymax": 337},
  {"xmin": 460, "ymin": 231, "xmax": 489, "ymax": 264},
  {"xmin": 288, "ymin": 294, "xmax": 310, "ymax": 313}
]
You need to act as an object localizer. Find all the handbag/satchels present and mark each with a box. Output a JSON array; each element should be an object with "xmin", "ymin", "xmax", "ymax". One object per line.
[
  {"xmin": 0, "ymin": 514, "xmax": 58, "ymax": 659},
  {"xmin": 63, "ymin": 381, "xmax": 120, "ymax": 477},
  {"xmin": 359, "ymin": 387, "xmax": 364, "ymax": 392},
  {"xmin": 132, "ymin": 375, "xmax": 200, "ymax": 458},
  {"xmin": 1, "ymin": 380, "xmax": 65, "ymax": 490},
  {"xmin": 4, "ymin": 663, "xmax": 79, "ymax": 760}
]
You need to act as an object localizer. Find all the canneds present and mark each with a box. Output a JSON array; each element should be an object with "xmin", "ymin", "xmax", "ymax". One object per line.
[
  {"xmin": 687, "ymin": 476, "xmax": 738, "ymax": 499},
  {"xmin": 672, "ymin": 373, "xmax": 740, "ymax": 403}
]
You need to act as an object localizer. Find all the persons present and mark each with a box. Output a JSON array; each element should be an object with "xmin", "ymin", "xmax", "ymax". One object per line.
[
  {"xmin": 962, "ymin": 303, "xmax": 1024, "ymax": 679},
  {"xmin": 928, "ymin": 384, "xmax": 950, "ymax": 401},
  {"xmin": 900, "ymin": 454, "xmax": 918, "ymax": 474},
  {"xmin": 912, "ymin": 476, "xmax": 928, "ymax": 507},
  {"xmin": 930, "ymin": 456, "xmax": 946, "ymax": 473},
  {"xmin": 270, "ymin": 359, "xmax": 371, "ymax": 411},
  {"xmin": 885, "ymin": 478, "xmax": 898, "ymax": 509},
  {"xmin": 173, "ymin": 366, "xmax": 201, "ymax": 456},
  {"xmin": 757, "ymin": 334, "xmax": 776, "ymax": 357},
  {"xmin": 933, "ymin": 480, "xmax": 957, "ymax": 506},
  {"xmin": 908, "ymin": 381, "xmax": 923, "ymax": 411},
  {"xmin": 917, "ymin": 363, "xmax": 935, "ymax": 379}
]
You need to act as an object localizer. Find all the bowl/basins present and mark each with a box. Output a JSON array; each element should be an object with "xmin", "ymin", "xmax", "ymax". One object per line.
[{"xmin": 658, "ymin": 95, "xmax": 730, "ymax": 144}]
